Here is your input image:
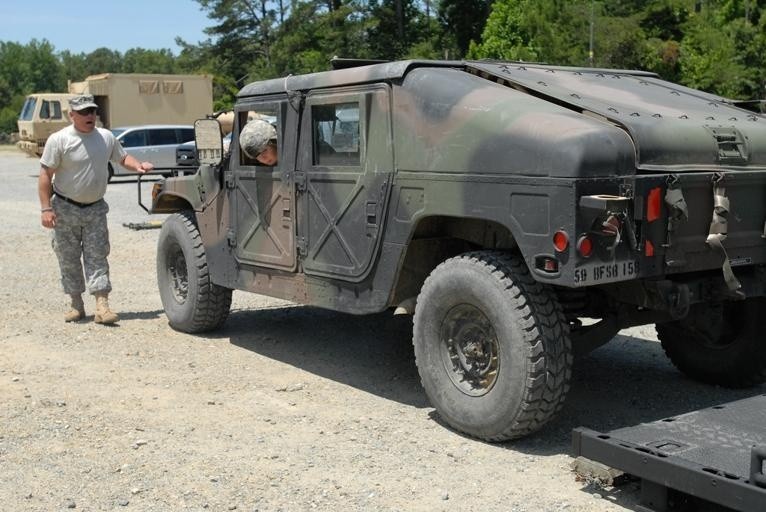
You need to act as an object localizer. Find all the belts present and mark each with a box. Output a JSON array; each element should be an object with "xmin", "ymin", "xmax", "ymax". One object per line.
[{"xmin": 55, "ymin": 192, "xmax": 103, "ymax": 208}]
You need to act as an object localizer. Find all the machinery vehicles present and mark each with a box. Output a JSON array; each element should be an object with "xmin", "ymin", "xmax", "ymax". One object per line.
[{"xmin": 139, "ymin": 59, "xmax": 766, "ymax": 444}]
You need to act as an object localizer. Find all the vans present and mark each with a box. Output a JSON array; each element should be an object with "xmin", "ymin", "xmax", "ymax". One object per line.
[{"xmin": 101, "ymin": 125, "xmax": 196, "ymax": 184}]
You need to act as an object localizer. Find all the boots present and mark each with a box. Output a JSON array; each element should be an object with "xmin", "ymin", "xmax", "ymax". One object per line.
[
  {"xmin": 94, "ymin": 292, "xmax": 120, "ymax": 323},
  {"xmin": 64, "ymin": 293, "xmax": 86, "ymax": 322}
]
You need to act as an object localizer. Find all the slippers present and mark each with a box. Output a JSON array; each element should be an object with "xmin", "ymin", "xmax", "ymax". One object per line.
[{"xmin": 77, "ymin": 110, "xmax": 95, "ymax": 116}]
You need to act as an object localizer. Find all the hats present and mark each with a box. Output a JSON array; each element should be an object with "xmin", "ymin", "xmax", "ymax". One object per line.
[{"xmin": 67, "ymin": 94, "xmax": 98, "ymax": 111}]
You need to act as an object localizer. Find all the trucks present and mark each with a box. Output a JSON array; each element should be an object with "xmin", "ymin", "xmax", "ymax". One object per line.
[{"xmin": 16, "ymin": 73, "xmax": 213, "ymax": 158}]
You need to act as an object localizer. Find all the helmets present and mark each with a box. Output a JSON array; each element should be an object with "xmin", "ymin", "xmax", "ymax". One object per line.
[{"xmin": 239, "ymin": 119, "xmax": 278, "ymax": 159}]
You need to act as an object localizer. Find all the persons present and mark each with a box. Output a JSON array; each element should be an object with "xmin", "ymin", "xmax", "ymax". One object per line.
[
  {"xmin": 239, "ymin": 121, "xmax": 278, "ymax": 166},
  {"xmin": 38, "ymin": 95, "xmax": 154, "ymax": 324}
]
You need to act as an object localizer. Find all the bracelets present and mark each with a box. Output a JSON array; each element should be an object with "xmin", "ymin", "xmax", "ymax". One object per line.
[{"xmin": 41, "ymin": 207, "xmax": 53, "ymax": 212}]
